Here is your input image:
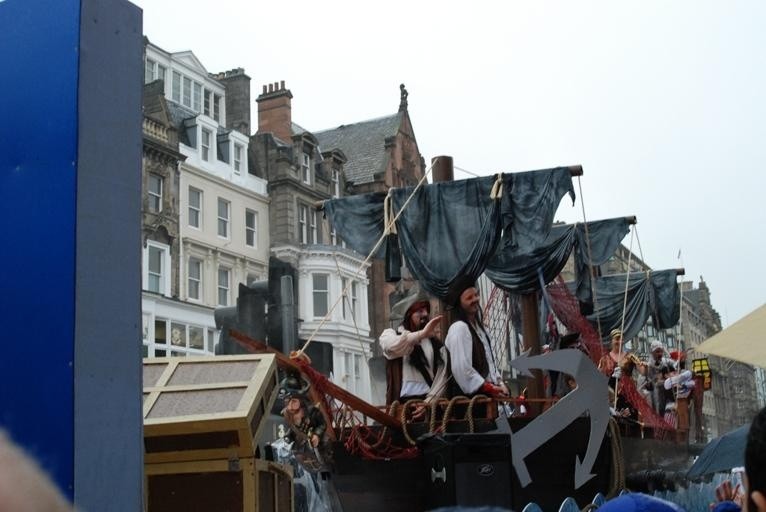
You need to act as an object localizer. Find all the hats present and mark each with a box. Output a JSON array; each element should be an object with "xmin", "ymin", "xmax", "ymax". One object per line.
[
  {"xmin": 650, "ymin": 341, "xmax": 664, "ymax": 351},
  {"xmin": 611, "ymin": 329, "xmax": 622, "ymax": 338},
  {"xmin": 393, "ymin": 293, "xmax": 430, "ymax": 317},
  {"xmin": 444, "ymin": 274, "xmax": 472, "ymax": 305}
]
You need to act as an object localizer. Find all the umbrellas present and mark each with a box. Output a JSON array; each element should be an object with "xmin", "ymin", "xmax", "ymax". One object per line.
[
  {"xmin": 695, "ymin": 302, "xmax": 766, "ymax": 370},
  {"xmin": 686, "ymin": 423, "xmax": 750, "ymax": 475}
]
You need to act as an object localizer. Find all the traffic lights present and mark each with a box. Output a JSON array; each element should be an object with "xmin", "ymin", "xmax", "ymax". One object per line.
[{"xmin": 213, "ymin": 285, "xmax": 269, "ymax": 354}]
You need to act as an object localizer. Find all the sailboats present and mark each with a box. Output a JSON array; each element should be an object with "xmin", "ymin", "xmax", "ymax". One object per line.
[{"xmin": 228, "ymin": 153, "xmax": 708, "ymax": 512}]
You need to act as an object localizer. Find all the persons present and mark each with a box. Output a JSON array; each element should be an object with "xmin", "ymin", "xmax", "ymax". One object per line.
[
  {"xmin": 596, "ymin": 479, "xmax": 744, "ymax": 512},
  {"xmin": 744, "ymin": 406, "xmax": 766, "ymax": 512},
  {"xmin": 542, "ymin": 329, "xmax": 695, "ymax": 440},
  {"xmin": 277, "ymin": 377, "xmax": 332, "ymax": 481},
  {"xmin": 379, "ymin": 295, "xmax": 444, "ymax": 421},
  {"xmin": 442, "ymin": 277, "xmax": 510, "ymax": 418}
]
